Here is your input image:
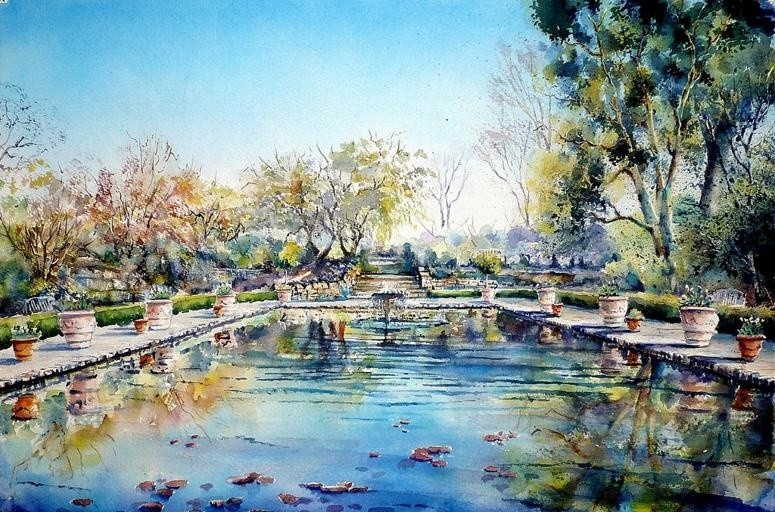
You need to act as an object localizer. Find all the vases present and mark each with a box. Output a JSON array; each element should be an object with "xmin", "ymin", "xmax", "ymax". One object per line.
[
  {"xmin": 550, "ymin": 327, "xmax": 562, "ymax": 340},
  {"xmin": 276, "ymin": 288, "xmax": 292, "ymax": 304},
  {"xmin": 482, "ymin": 288, "xmax": 495, "ymax": 303},
  {"xmin": 139, "ymin": 353, "xmax": 154, "ymax": 370},
  {"xmin": 551, "ymin": 303, "xmax": 564, "ymax": 317},
  {"xmin": 214, "ymin": 330, "xmax": 231, "ymax": 343},
  {"xmin": 213, "ymin": 306, "xmax": 224, "ymax": 318},
  {"xmin": 133, "ymin": 319, "xmax": 149, "ymax": 333}
]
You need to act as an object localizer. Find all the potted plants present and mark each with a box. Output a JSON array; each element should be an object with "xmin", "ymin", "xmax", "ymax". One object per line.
[
  {"xmin": 736, "ymin": 315, "xmax": 767, "ymax": 362},
  {"xmin": 598, "ymin": 286, "xmax": 629, "ymax": 328},
  {"xmin": 674, "ymin": 382, "xmax": 720, "ymax": 435},
  {"xmin": 66, "ymin": 373, "xmax": 101, "ymax": 417},
  {"xmin": 538, "ymin": 325, "xmax": 563, "ymax": 346},
  {"xmin": 10, "ymin": 328, "xmax": 42, "ymax": 362},
  {"xmin": 727, "ymin": 385, "xmax": 757, "ymax": 430},
  {"xmin": 58, "ymin": 290, "xmax": 97, "ymax": 350},
  {"xmin": 601, "ymin": 342, "xmax": 624, "ymax": 375},
  {"xmin": 13, "ymin": 393, "xmax": 40, "ymax": 427},
  {"xmin": 532, "ymin": 277, "xmax": 569, "ymax": 314},
  {"xmin": 625, "ymin": 309, "xmax": 645, "ymax": 332},
  {"xmin": 146, "ymin": 290, "xmax": 173, "ymax": 330},
  {"xmin": 625, "ymin": 350, "xmax": 641, "ymax": 368},
  {"xmin": 678, "ymin": 284, "xmax": 720, "ymax": 348},
  {"xmin": 216, "ymin": 286, "xmax": 236, "ymax": 316},
  {"xmin": 220, "ymin": 329, "xmax": 239, "ymax": 349},
  {"xmin": 151, "ymin": 347, "xmax": 181, "ymax": 375}
]
